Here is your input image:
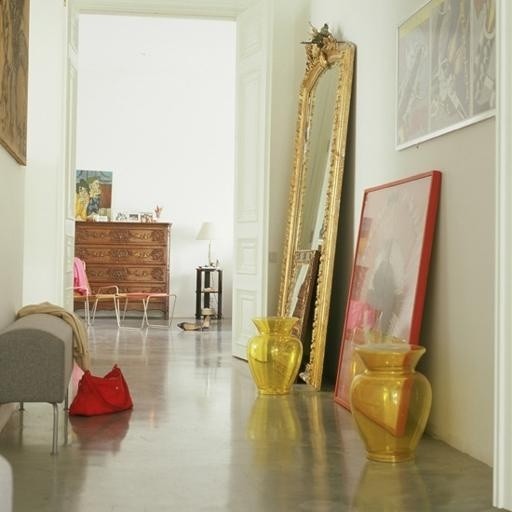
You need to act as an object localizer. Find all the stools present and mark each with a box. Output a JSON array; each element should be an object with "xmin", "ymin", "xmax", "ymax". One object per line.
[{"xmin": 72, "ymin": 286, "xmax": 177, "ymax": 330}]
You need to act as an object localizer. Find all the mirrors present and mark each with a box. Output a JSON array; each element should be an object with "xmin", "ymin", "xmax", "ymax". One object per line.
[{"xmin": 276, "ymin": 19, "xmax": 360, "ymax": 391}]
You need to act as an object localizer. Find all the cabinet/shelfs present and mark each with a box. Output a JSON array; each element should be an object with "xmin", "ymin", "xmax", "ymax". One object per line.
[
  {"xmin": 195, "ymin": 268, "xmax": 223, "ymax": 321},
  {"xmin": 73, "ymin": 222, "xmax": 172, "ymax": 321}
]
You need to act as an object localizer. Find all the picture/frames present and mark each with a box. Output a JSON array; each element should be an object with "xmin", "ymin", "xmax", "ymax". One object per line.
[
  {"xmin": 392, "ymin": 4, "xmax": 496, "ymax": 153},
  {"xmin": 280, "ymin": 248, "xmax": 321, "ymax": 371},
  {"xmin": 330, "ymin": 166, "xmax": 446, "ymax": 436}
]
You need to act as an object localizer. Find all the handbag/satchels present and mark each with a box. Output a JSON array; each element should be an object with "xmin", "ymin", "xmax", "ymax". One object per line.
[{"xmin": 68, "ymin": 364, "xmax": 133, "ymax": 417}]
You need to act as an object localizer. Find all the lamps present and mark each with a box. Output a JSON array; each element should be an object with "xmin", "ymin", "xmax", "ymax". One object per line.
[{"xmin": 196, "ymin": 222, "xmax": 223, "ymax": 267}]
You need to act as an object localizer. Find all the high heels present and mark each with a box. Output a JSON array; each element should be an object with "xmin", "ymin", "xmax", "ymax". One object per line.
[
  {"xmin": 176, "ymin": 322, "xmax": 203, "ymax": 331},
  {"xmin": 201, "ymin": 315, "xmax": 212, "ymax": 329}
]
